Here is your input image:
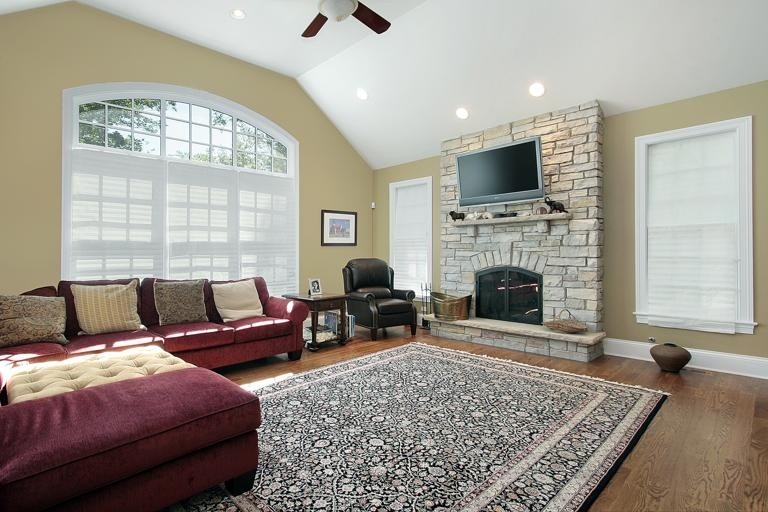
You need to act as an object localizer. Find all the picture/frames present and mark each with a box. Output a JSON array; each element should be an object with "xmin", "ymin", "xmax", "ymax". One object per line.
[
  {"xmin": 308, "ymin": 278, "xmax": 322, "ymax": 295},
  {"xmin": 321, "ymin": 209, "xmax": 357, "ymax": 246}
]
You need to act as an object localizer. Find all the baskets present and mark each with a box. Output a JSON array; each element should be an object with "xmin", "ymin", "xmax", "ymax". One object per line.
[{"xmin": 540, "ymin": 308, "xmax": 589, "ymax": 335}]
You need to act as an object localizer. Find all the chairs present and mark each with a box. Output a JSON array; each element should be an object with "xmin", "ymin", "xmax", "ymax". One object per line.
[{"xmin": 343, "ymin": 258, "xmax": 417, "ymax": 341}]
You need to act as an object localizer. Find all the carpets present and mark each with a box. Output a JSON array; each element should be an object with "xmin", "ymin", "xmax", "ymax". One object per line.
[{"xmin": 159, "ymin": 341, "xmax": 671, "ymax": 512}]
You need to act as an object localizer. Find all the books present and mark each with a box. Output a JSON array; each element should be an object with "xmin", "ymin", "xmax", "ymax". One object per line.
[{"xmin": 323, "ymin": 312, "xmax": 357, "ymax": 341}]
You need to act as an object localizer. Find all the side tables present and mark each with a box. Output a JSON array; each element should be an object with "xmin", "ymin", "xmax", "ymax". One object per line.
[{"xmin": 282, "ymin": 291, "xmax": 352, "ymax": 351}]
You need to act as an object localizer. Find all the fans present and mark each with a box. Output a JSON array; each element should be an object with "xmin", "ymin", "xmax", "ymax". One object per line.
[{"xmin": 301, "ymin": 0, "xmax": 391, "ymax": 37}]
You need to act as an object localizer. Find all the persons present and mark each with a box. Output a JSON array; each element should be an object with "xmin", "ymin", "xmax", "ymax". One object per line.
[{"xmin": 312, "ymin": 281, "xmax": 320, "ymax": 292}]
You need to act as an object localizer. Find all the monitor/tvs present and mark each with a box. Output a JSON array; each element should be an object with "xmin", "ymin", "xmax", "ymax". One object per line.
[{"xmin": 455, "ymin": 137, "xmax": 545, "ymax": 208}]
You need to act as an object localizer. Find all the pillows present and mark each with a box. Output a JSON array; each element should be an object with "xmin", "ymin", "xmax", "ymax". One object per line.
[
  {"xmin": 154, "ymin": 280, "xmax": 209, "ymax": 326},
  {"xmin": 211, "ymin": 279, "xmax": 266, "ymax": 322},
  {"xmin": 0, "ymin": 295, "xmax": 69, "ymax": 348},
  {"xmin": 70, "ymin": 279, "xmax": 147, "ymax": 335}
]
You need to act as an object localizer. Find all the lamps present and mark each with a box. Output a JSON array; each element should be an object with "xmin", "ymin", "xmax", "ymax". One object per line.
[{"xmin": 317, "ymin": 0, "xmax": 358, "ymax": 22}]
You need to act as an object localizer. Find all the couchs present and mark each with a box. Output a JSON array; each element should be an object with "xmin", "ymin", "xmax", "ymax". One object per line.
[{"xmin": 0, "ymin": 276, "xmax": 308, "ymax": 512}]
